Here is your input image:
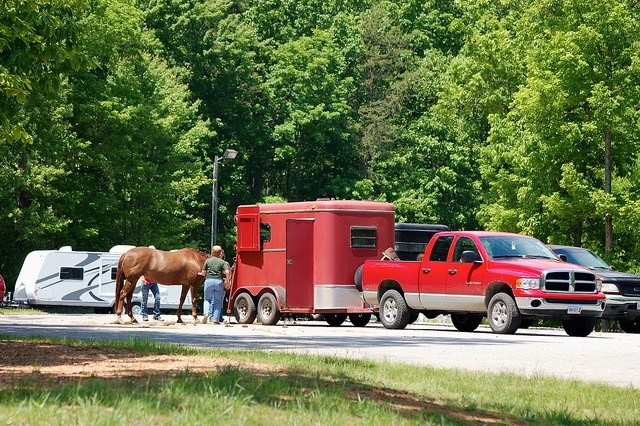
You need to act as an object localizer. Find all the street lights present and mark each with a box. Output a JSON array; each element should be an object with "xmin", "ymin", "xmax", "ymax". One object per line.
[{"xmin": 211, "ymin": 148, "xmax": 237, "ymax": 246}]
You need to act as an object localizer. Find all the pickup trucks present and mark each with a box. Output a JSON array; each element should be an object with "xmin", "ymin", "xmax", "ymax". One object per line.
[{"xmin": 362, "ymin": 231, "xmax": 606, "ymax": 337}]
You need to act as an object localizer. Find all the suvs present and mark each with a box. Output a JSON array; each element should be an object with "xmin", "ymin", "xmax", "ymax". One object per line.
[{"xmin": 548, "ymin": 245, "xmax": 640, "ymax": 333}]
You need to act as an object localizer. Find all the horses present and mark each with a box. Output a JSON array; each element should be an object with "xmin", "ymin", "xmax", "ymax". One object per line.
[{"xmin": 115, "ymin": 246, "xmax": 233, "ymax": 325}]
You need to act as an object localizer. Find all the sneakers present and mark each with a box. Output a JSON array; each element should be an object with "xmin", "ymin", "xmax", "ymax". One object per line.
[
  {"xmin": 142, "ymin": 315, "xmax": 148, "ymax": 321},
  {"xmin": 213, "ymin": 320, "xmax": 221, "ymax": 325},
  {"xmin": 209, "ymin": 316, "xmax": 214, "ymax": 322},
  {"xmin": 154, "ymin": 316, "xmax": 164, "ymax": 321},
  {"xmin": 219, "ymin": 317, "xmax": 227, "ymax": 322},
  {"xmin": 202, "ymin": 315, "xmax": 208, "ymax": 324}
]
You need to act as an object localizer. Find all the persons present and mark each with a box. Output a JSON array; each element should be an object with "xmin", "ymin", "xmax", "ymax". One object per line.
[
  {"xmin": 201, "ymin": 245, "xmax": 229, "ymax": 324},
  {"xmin": 205, "ymin": 248, "xmax": 231, "ymax": 322},
  {"xmin": 416, "ymin": 254, "xmax": 424, "ymax": 261},
  {"xmin": 142, "ymin": 246, "xmax": 164, "ymax": 321}
]
